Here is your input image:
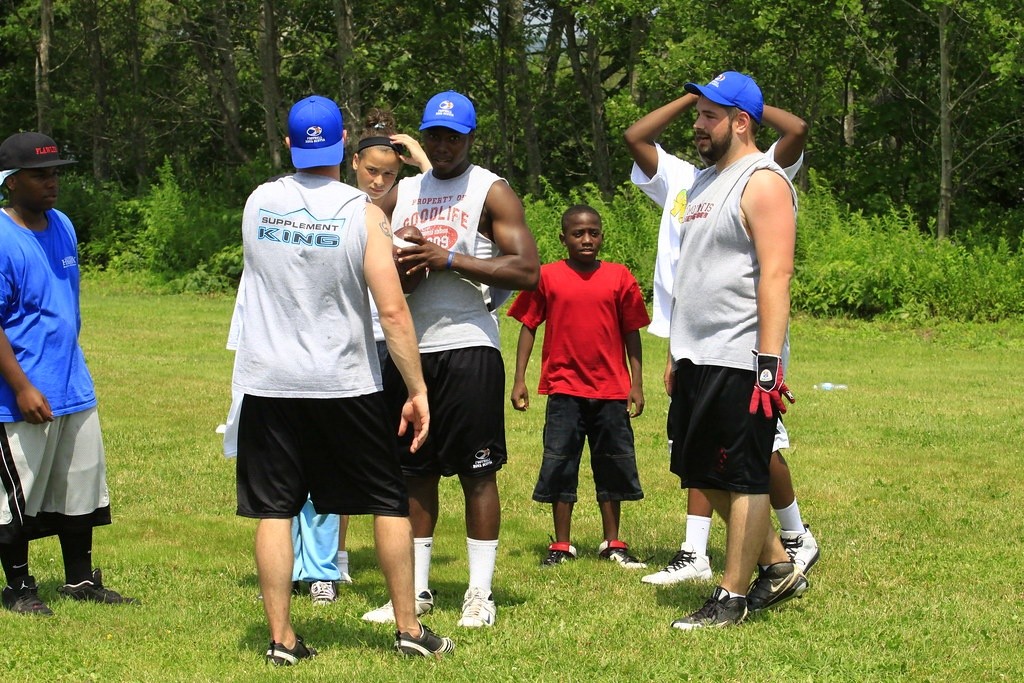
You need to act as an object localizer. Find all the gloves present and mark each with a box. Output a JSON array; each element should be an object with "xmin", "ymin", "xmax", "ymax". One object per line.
[{"xmin": 749, "ymin": 348, "xmax": 796, "ymax": 418}]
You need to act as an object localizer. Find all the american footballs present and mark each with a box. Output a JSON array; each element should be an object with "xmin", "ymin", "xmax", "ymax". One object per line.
[{"xmin": 390, "ymin": 224, "xmax": 425, "ymax": 294}]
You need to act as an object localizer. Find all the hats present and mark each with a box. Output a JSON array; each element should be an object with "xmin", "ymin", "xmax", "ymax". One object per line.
[
  {"xmin": 288, "ymin": 95, "xmax": 343, "ymax": 168},
  {"xmin": 420, "ymin": 90, "xmax": 477, "ymax": 134},
  {"xmin": 0, "ymin": 133, "xmax": 79, "ymax": 168},
  {"xmin": 685, "ymin": 70, "xmax": 764, "ymax": 122}
]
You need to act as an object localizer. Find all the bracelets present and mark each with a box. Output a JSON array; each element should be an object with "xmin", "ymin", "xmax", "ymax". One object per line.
[{"xmin": 447, "ymin": 252, "xmax": 454, "ymax": 269}]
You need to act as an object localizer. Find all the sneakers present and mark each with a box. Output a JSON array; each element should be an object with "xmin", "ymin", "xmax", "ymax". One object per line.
[
  {"xmin": 292, "ymin": 581, "xmax": 300, "ymax": 596},
  {"xmin": 671, "ymin": 586, "xmax": 748, "ymax": 631},
  {"xmin": 57, "ymin": 568, "xmax": 141, "ymax": 607},
  {"xmin": 1, "ymin": 575, "xmax": 55, "ymax": 616},
  {"xmin": 265, "ymin": 634, "xmax": 318, "ymax": 668},
  {"xmin": 393, "ymin": 620, "xmax": 455, "ymax": 661},
  {"xmin": 640, "ymin": 542, "xmax": 713, "ymax": 584},
  {"xmin": 779, "ymin": 523, "xmax": 821, "ymax": 576},
  {"xmin": 361, "ymin": 589, "xmax": 437, "ymax": 622},
  {"xmin": 457, "ymin": 587, "xmax": 497, "ymax": 628},
  {"xmin": 309, "ymin": 579, "xmax": 338, "ymax": 608},
  {"xmin": 746, "ymin": 552, "xmax": 810, "ymax": 615},
  {"xmin": 598, "ymin": 540, "xmax": 649, "ymax": 570},
  {"xmin": 541, "ymin": 534, "xmax": 578, "ymax": 572}
]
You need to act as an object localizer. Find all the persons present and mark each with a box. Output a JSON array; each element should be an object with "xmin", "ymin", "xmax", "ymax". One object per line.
[
  {"xmin": 0, "ymin": 131, "xmax": 134, "ymax": 617},
  {"xmin": 223, "ymin": 95, "xmax": 454, "ymax": 665},
  {"xmin": 624, "ymin": 92, "xmax": 820, "ymax": 587},
  {"xmin": 336, "ymin": 109, "xmax": 433, "ymax": 581},
  {"xmin": 509, "ymin": 205, "xmax": 650, "ymax": 570},
  {"xmin": 359, "ymin": 90, "xmax": 541, "ymax": 628},
  {"xmin": 662, "ymin": 71, "xmax": 809, "ymax": 631},
  {"xmin": 290, "ymin": 494, "xmax": 340, "ymax": 608}
]
